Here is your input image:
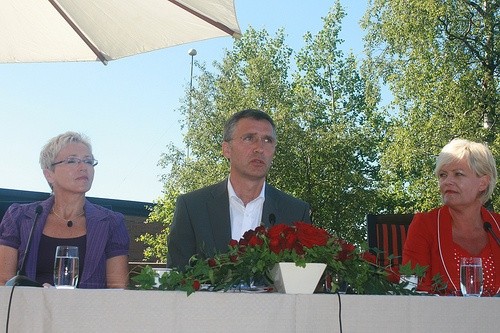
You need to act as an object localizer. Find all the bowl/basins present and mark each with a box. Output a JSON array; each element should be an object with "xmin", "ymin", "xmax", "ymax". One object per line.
[
  {"xmin": 145, "ymin": 267, "xmax": 178, "ymax": 288},
  {"xmin": 387, "ymin": 275, "xmax": 418, "ymax": 294}
]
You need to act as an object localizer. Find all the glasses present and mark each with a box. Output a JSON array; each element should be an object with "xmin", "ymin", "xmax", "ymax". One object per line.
[
  {"xmin": 49, "ymin": 158, "xmax": 98, "ymax": 167},
  {"xmin": 227, "ymin": 135, "xmax": 277, "ymax": 147}
]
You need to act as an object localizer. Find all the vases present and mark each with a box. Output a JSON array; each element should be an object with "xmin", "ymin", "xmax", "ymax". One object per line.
[{"xmin": 268, "ymin": 262, "xmax": 327, "ymax": 295}]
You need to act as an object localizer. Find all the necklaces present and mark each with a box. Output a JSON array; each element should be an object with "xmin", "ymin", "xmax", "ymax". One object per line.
[{"xmin": 53, "ymin": 206, "xmax": 85, "ymax": 227}]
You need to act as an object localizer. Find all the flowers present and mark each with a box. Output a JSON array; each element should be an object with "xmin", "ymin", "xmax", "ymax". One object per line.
[{"xmin": 130, "ymin": 221, "xmax": 449, "ymax": 296}]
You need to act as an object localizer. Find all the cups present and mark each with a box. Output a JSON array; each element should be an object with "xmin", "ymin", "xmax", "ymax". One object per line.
[
  {"xmin": 460, "ymin": 257, "xmax": 483, "ymax": 297},
  {"xmin": 53, "ymin": 246, "xmax": 80, "ymax": 289}
]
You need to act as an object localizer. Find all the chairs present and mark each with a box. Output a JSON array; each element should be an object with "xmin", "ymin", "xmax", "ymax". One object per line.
[{"xmin": 367, "ymin": 214, "xmax": 415, "ymax": 276}]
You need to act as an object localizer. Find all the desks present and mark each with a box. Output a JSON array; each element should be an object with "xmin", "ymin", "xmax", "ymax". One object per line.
[{"xmin": 0, "ymin": 286, "xmax": 500, "ymax": 333}]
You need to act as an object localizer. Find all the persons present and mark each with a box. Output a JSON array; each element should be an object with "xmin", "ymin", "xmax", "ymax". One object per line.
[
  {"xmin": 402, "ymin": 138, "xmax": 500, "ymax": 296},
  {"xmin": 0, "ymin": 130, "xmax": 130, "ymax": 289},
  {"xmin": 167, "ymin": 109, "xmax": 312, "ymax": 270}
]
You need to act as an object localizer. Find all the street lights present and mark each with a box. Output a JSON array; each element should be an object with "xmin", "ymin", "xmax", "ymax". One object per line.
[{"xmin": 188, "ymin": 49, "xmax": 197, "ymax": 160}]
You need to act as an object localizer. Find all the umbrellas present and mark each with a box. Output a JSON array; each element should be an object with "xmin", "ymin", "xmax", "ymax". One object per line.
[{"xmin": 0, "ymin": 0, "xmax": 243, "ymax": 65}]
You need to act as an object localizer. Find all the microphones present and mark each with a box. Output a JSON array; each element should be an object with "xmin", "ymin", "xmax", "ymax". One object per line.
[
  {"xmin": 5, "ymin": 206, "xmax": 44, "ymax": 287},
  {"xmin": 484, "ymin": 222, "xmax": 500, "ymax": 247},
  {"xmin": 269, "ymin": 213, "xmax": 276, "ymax": 226}
]
